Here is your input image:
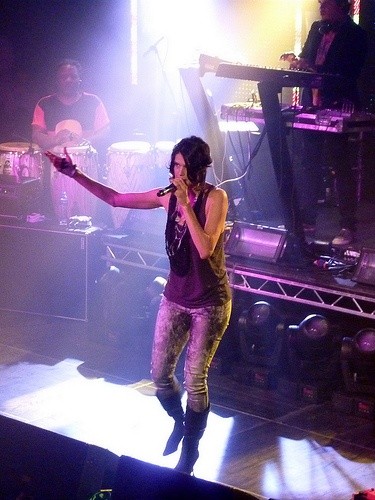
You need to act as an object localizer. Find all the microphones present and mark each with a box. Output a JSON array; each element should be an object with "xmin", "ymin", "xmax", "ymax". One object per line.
[{"xmin": 157, "ymin": 180, "xmax": 186, "ymax": 196}]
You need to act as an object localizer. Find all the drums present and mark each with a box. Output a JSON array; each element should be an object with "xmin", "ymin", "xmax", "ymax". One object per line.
[
  {"xmin": 0, "ymin": 142, "xmax": 43, "ymax": 196},
  {"xmin": 49, "ymin": 144, "xmax": 99, "ymax": 223},
  {"xmin": 105, "ymin": 141, "xmax": 155, "ymax": 228}
]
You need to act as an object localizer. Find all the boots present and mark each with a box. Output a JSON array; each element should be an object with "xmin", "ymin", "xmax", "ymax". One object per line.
[
  {"xmin": 173, "ymin": 404, "xmax": 212, "ymax": 473},
  {"xmin": 156, "ymin": 383, "xmax": 186, "ymax": 456}
]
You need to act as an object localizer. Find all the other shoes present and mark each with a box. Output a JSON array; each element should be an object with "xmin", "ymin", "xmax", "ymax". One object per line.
[
  {"xmin": 331, "ymin": 228, "xmax": 355, "ymax": 246},
  {"xmin": 278, "ymin": 221, "xmax": 318, "ymax": 233}
]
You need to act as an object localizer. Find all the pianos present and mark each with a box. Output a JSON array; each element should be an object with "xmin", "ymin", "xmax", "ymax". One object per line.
[{"xmin": 214, "ymin": 62, "xmax": 375, "ymax": 225}]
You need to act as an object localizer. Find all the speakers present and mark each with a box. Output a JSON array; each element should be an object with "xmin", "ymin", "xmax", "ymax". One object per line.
[{"xmin": 0, "ymin": 221, "xmax": 105, "ymax": 322}]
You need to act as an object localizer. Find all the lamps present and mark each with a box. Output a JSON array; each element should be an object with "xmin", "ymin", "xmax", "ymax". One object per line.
[
  {"xmin": 276, "ymin": 315, "xmax": 342, "ymax": 405},
  {"xmin": 332, "ymin": 328, "xmax": 375, "ymax": 417},
  {"xmin": 223, "ymin": 301, "xmax": 285, "ymax": 390},
  {"xmin": 92, "ymin": 265, "xmax": 168, "ymax": 355},
  {"xmin": 351, "ymin": 247, "xmax": 375, "ymax": 285},
  {"xmin": 227, "ymin": 222, "xmax": 290, "ymax": 263}
]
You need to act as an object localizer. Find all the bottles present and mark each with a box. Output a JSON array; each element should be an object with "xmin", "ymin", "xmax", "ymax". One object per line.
[{"xmin": 58, "ymin": 192, "xmax": 67, "ymax": 225}]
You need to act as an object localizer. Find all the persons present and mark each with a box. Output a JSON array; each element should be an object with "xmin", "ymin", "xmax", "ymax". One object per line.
[
  {"xmin": 43, "ymin": 135, "xmax": 234, "ymax": 472},
  {"xmin": 277, "ymin": 0, "xmax": 375, "ymax": 246},
  {"xmin": 32, "ymin": 59, "xmax": 111, "ymax": 150}
]
